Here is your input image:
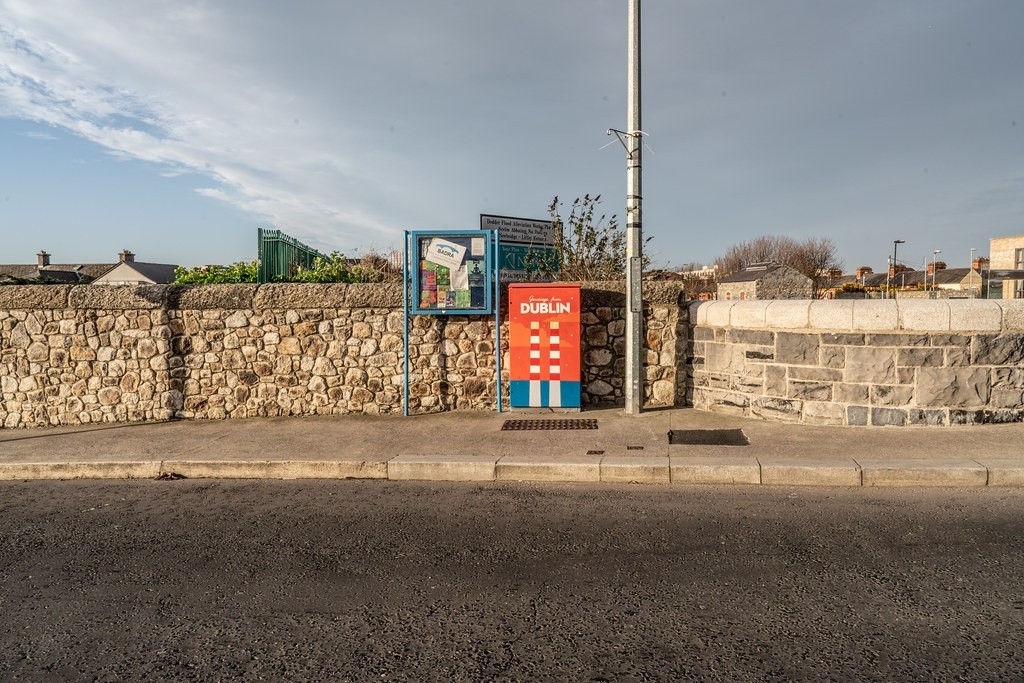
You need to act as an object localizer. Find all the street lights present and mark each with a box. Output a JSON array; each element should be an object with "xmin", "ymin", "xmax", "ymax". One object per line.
[
  {"xmin": 969, "ymin": 247, "xmax": 976, "ymax": 287},
  {"xmin": 932, "ymin": 250, "xmax": 941, "ymax": 290},
  {"xmin": 893, "ymin": 239, "xmax": 906, "ymax": 298}
]
